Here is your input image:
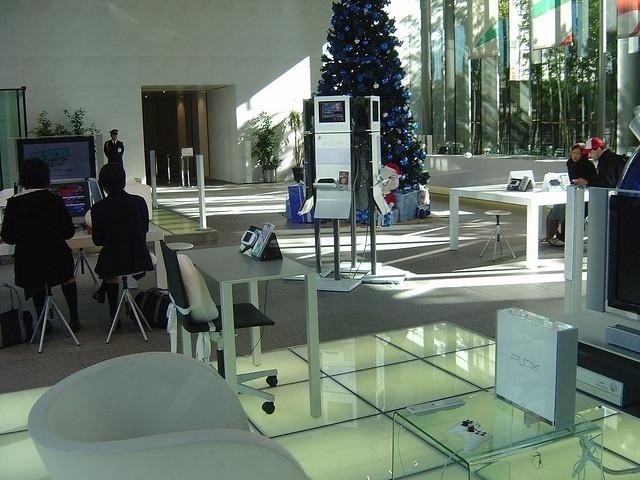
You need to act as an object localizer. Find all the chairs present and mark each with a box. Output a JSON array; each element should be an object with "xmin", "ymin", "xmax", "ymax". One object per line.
[
  {"xmin": 28, "ymin": 351, "xmax": 312, "ymax": 480},
  {"xmin": 158, "ymin": 239, "xmax": 280, "ymax": 414}
]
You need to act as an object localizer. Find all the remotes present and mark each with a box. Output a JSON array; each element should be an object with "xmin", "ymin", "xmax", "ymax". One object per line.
[{"xmin": 406, "ymin": 397, "xmax": 465, "ymax": 415}]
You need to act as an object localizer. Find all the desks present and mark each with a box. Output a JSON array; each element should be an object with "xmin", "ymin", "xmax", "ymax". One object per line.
[
  {"xmin": 0, "ymin": 224, "xmax": 168, "ymax": 291},
  {"xmin": 450, "ymin": 181, "xmax": 589, "ymax": 272},
  {"xmin": 164, "ymin": 234, "xmax": 322, "ymax": 418}
]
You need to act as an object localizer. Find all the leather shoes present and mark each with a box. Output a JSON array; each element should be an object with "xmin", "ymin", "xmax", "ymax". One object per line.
[{"xmin": 94, "ymin": 290, "xmax": 105, "ymax": 303}]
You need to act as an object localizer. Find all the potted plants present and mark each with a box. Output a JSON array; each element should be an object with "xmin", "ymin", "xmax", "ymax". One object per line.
[
  {"xmin": 248, "ymin": 112, "xmax": 286, "ymax": 183},
  {"xmin": 287, "ymin": 110, "xmax": 305, "ymax": 181}
]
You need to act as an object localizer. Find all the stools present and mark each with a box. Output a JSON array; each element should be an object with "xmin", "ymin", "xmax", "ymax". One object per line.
[
  {"xmin": 479, "ymin": 210, "xmax": 516, "ymax": 261},
  {"xmin": 106, "ymin": 270, "xmax": 153, "ymax": 343},
  {"xmin": 30, "ymin": 277, "xmax": 81, "ymax": 354}
]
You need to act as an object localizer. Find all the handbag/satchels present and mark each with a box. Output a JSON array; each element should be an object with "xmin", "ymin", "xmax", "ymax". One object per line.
[
  {"xmin": 0, "ymin": 309, "xmax": 34, "ymax": 348},
  {"xmin": 129, "ymin": 288, "xmax": 167, "ymax": 329}
]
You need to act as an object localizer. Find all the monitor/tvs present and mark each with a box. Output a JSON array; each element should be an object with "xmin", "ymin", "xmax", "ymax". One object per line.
[
  {"xmin": 604, "ymin": 189, "xmax": 640, "ymax": 323},
  {"xmin": 314, "ymin": 95, "xmax": 352, "ymax": 133},
  {"xmin": 16, "ymin": 135, "xmax": 96, "ymax": 185}
]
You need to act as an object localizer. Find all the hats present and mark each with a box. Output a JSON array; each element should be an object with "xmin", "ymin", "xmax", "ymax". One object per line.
[
  {"xmin": 582, "ymin": 137, "xmax": 603, "ymax": 154},
  {"xmin": 109, "ymin": 129, "xmax": 118, "ymax": 135}
]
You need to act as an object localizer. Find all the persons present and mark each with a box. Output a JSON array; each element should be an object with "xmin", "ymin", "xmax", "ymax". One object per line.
[
  {"xmin": 1, "ymin": 157, "xmax": 81, "ymax": 335},
  {"xmin": 549, "ymin": 144, "xmax": 597, "ymax": 246},
  {"xmin": 572, "ymin": 137, "xmax": 626, "ymax": 188},
  {"xmin": 104, "ymin": 129, "xmax": 125, "ymax": 166},
  {"xmin": 91, "ymin": 164, "xmax": 154, "ymax": 328}
]
